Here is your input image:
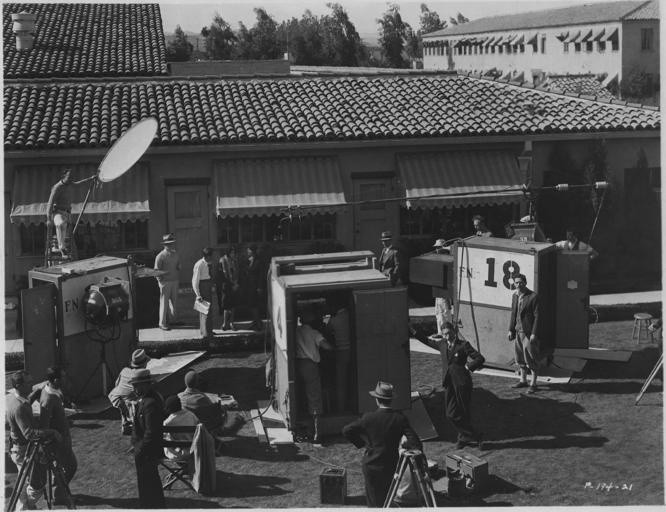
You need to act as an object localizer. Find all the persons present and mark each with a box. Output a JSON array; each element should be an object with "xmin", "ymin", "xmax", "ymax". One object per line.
[
  {"xmin": 39, "ymin": 366, "xmax": 78, "ymax": 506},
  {"xmin": 438, "ymin": 322, "xmax": 484, "ymax": 451},
  {"xmin": 340, "ymin": 381, "xmax": 422, "ymax": 507},
  {"xmin": 508, "ymin": 274, "xmax": 543, "ymax": 395},
  {"xmin": 430, "ymin": 238, "xmax": 452, "ymax": 338},
  {"xmin": 378, "ymin": 230, "xmax": 401, "ymax": 286},
  {"xmin": 471, "ymin": 213, "xmax": 493, "ymax": 238},
  {"xmin": 45, "ymin": 167, "xmax": 98, "ymax": 257},
  {"xmin": 107, "ymin": 234, "xmax": 354, "ymax": 509},
  {"xmin": 5, "ymin": 369, "xmax": 61, "ymax": 510},
  {"xmin": 554, "ymin": 227, "xmax": 599, "ymax": 260}
]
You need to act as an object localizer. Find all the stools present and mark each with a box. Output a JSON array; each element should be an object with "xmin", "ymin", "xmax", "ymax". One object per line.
[{"xmin": 632, "ymin": 312, "xmax": 654, "ymax": 345}]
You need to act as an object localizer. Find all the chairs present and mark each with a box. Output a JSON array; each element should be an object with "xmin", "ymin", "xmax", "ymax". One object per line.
[
  {"xmin": 157, "ymin": 423, "xmax": 203, "ymax": 491},
  {"xmin": 183, "ymin": 399, "xmax": 221, "ymax": 441}
]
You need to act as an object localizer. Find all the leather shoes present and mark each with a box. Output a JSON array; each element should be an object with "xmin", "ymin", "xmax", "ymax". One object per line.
[
  {"xmin": 158, "ymin": 324, "xmax": 171, "ymax": 331},
  {"xmin": 172, "ymin": 321, "xmax": 185, "ymax": 326},
  {"xmin": 453, "ymin": 439, "xmax": 467, "ymax": 450}
]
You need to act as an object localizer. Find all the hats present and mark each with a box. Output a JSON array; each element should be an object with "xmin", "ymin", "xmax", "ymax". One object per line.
[
  {"xmin": 431, "ymin": 238, "xmax": 447, "ymax": 248},
  {"xmin": 127, "ymin": 369, "xmax": 157, "ymax": 385},
  {"xmin": 520, "ymin": 214, "xmax": 535, "ymax": 223},
  {"xmin": 130, "ymin": 348, "xmax": 152, "ymax": 368},
  {"xmin": 379, "ymin": 230, "xmax": 394, "ymax": 242},
  {"xmin": 159, "ymin": 232, "xmax": 178, "ymax": 246},
  {"xmin": 245, "ymin": 243, "xmax": 259, "ymax": 249},
  {"xmin": 367, "ymin": 379, "xmax": 399, "ymax": 401},
  {"xmin": 184, "ymin": 369, "xmax": 199, "ymax": 388}
]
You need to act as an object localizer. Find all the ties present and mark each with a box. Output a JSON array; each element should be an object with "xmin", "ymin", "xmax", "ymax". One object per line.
[
  {"xmin": 448, "ymin": 341, "xmax": 454, "ymax": 350},
  {"xmin": 208, "ymin": 265, "xmax": 215, "ymax": 281}
]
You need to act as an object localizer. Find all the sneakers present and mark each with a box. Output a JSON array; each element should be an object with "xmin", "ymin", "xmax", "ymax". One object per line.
[
  {"xmin": 525, "ymin": 383, "xmax": 538, "ymax": 395},
  {"xmin": 221, "ymin": 324, "xmax": 228, "ymax": 331},
  {"xmin": 231, "ymin": 325, "xmax": 237, "ymax": 331},
  {"xmin": 511, "ymin": 381, "xmax": 529, "ymax": 389},
  {"xmin": 432, "ymin": 334, "xmax": 443, "ymax": 338},
  {"xmin": 52, "ymin": 494, "xmax": 78, "ymax": 506}
]
